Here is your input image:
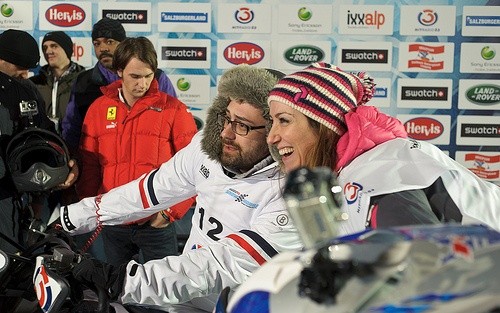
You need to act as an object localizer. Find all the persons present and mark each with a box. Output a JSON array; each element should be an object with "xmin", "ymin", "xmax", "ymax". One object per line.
[
  {"xmin": 267, "ymin": 62, "xmax": 499, "ymax": 237},
  {"xmin": 60, "ymin": 66, "xmax": 303, "ymax": 313},
  {"xmin": 0, "ymin": 17, "xmax": 199, "ymax": 313}
]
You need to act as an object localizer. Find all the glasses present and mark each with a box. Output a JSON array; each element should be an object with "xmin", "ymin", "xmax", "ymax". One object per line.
[{"xmin": 216, "ymin": 111, "xmax": 266, "ymax": 135}]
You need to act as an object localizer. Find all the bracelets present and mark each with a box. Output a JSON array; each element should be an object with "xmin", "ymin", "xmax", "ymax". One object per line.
[{"xmin": 160, "ymin": 210, "xmax": 169, "ymax": 221}]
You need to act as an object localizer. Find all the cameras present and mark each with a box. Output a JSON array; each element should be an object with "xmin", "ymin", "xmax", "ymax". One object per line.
[
  {"xmin": 282, "ymin": 166, "xmax": 347, "ymax": 249},
  {"xmin": 18, "ymin": 99, "xmax": 38, "ymax": 117}
]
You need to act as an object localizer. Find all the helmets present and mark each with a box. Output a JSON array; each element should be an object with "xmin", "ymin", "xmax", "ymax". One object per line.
[{"xmin": 5, "ymin": 125, "xmax": 72, "ymax": 194}]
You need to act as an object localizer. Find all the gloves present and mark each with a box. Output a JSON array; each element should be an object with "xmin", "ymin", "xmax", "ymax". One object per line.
[
  {"xmin": 44, "ymin": 226, "xmax": 70, "ymax": 249},
  {"xmin": 67, "ymin": 248, "xmax": 126, "ymax": 307}
]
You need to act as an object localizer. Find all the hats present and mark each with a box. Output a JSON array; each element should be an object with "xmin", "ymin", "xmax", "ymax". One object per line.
[
  {"xmin": 92, "ymin": 17, "xmax": 126, "ymax": 42},
  {"xmin": 268, "ymin": 62, "xmax": 376, "ymax": 138},
  {"xmin": 42, "ymin": 30, "xmax": 73, "ymax": 59},
  {"xmin": 0, "ymin": 28, "xmax": 40, "ymax": 68}
]
